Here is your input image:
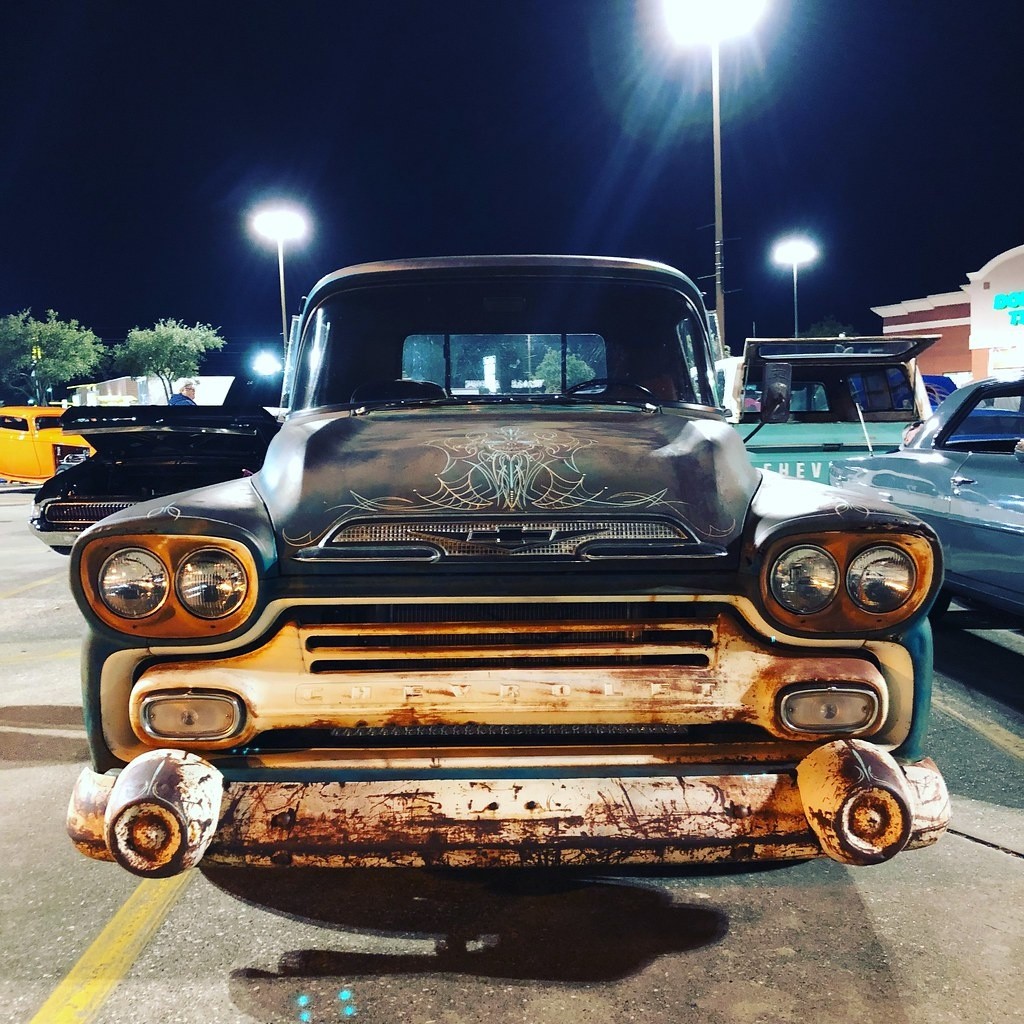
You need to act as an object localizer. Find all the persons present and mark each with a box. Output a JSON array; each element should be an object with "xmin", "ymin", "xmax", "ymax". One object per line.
[
  {"xmin": 168, "ymin": 378, "xmax": 198, "ymax": 406},
  {"xmin": 885, "ymin": 423, "xmax": 924, "ymax": 454}
]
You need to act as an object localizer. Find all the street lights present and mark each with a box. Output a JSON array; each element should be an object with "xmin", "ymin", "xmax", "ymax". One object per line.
[
  {"xmin": 772, "ymin": 234, "xmax": 818, "ymax": 339},
  {"xmin": 252, "ymin": 202, "xmax": 306, "ymax": 356},
  {"xmin": 664, "ymin": 0, "xmax": 770, "ymax": 354}
]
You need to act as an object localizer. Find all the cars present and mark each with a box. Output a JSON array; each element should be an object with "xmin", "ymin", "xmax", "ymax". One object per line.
[
  {"xmin": 826, "ymin": 375, "xmax": 1024, "ymax": 614},
  {"xmin": 1, "ymin": 405, "xmax": 97, "ymax": 485},
  {"xmin": 66, "ymin": 253, "xmax": 955, "ymax": 871},
  {"xmin": 736, "ymin": 334, "xmax": 932, "ymax": 490},
  {"xmin": 33, "ymin": 349, "xmax": 284, "ymax": 553}
]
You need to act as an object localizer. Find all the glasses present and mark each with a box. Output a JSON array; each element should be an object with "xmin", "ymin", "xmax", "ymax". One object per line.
[{"xmin": 185, "ymin": 387, "xmax": 194, "ymax": 392}]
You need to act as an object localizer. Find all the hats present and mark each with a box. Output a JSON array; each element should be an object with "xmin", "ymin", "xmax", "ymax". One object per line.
[{"xmin": 173, "ymin": 377, "xmax": 193, "ymax": 394}]
